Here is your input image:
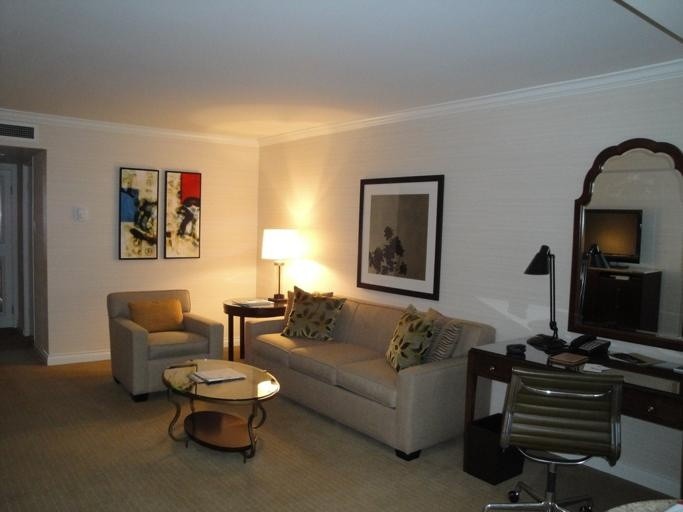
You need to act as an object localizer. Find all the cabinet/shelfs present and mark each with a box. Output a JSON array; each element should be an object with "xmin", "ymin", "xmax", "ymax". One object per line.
[{"xmin": 585, "ymin": 270, "xmax": 662, "ymax": 332}]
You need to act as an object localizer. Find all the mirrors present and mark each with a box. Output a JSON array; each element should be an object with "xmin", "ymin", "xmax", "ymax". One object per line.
[{"xmin": 568, "ymin": 137, "xmax": 682, "ymax": 351}]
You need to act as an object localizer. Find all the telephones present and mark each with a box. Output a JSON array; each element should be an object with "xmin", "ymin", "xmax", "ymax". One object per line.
[{"xmin": 568, "ymin": 332, "xmax": 611, "ymax": 356}]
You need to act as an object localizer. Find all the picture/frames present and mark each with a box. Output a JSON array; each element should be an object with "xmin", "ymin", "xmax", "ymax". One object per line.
[
  {"xmin": 164, "ymin": 170, "xmax": 202, "ymax": 259},
  {"xmin": 119, "ymin": 166, "xmax": 159, "ymax": 260},
  {"xmin": 356, "ymin": 174, "xmax": 444, "ymax": 301}
]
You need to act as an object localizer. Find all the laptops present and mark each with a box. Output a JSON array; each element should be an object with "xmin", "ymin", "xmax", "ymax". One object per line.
[{"xmin": 187, "ymin": 367, "xmax": 247, "ymax": 385}]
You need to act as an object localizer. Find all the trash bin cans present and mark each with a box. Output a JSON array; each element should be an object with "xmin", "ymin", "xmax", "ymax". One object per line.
[{"xmin": 463, "ymin": 413, "xmax": 525, "ymax": 485}]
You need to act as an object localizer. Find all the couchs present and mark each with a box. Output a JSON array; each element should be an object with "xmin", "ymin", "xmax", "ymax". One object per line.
[
  {"xmin": 108, "ymin": 287, "xmax": 224, "ymax": 401},
  {"xmin": 244, "ymin": 296, "xmax": 494, "ymax": 461}
]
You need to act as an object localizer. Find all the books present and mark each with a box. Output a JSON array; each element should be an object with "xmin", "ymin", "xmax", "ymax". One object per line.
[
  {"xmin": 548, "ymin": 352, "xmax": 588, "ymax": 366},
  {"xmin": 186, "ymin": 368, "xmax": 247, "ymax": 384},
  {"xmin": 545, "ymin": 362, "xmax": 584, "ymax": 371}
]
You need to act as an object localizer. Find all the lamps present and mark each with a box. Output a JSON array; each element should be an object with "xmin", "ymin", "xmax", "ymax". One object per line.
[
  {"xmin": 261, "ymin": 229, "xmax": 301, "ymax": 303},
  {"xmin": 579, "ymin": 243, "xmax": 608, "ymax": 315},
  {"xmin": 524, "ymin": 244, "xmax": 566, "ymax": 351}
]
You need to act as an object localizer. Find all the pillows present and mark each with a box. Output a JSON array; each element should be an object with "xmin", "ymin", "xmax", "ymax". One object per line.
[
  {"xmin": 425, "ymin": 308, "xmax": 463, "ymax": 364},
  {"xmin": 285, "ymin": 291, "xmax": 332, "ymax": 324},
  {"xmin": 127, "ymin": 300, "xmax": 182, "ymax": 330},
  {"xmin": 385, "ymin": 304, "xmax": 434, "ymax": 375},
  {"xmin": 281, "ymin": 286, "xmax": 346, "ymax": 342}
]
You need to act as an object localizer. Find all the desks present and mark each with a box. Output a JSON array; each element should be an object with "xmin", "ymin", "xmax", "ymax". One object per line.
[
  {"xmin": 464, "ymin": 336, "xmax": 683, "ymax": 485},
  {"xmin": 223, "ymin": 299, "xmax": 285, "ymax": 360}
]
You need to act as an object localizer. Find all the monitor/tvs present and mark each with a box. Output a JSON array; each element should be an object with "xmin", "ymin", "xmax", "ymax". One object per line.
[{"xmin": 584, "ymin": 209, "xmax": 643, "ymax": 269}]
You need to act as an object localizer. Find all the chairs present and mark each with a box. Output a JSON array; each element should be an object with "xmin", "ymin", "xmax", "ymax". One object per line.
[{"xmin": 482, "ymin": 366, "xmax": 623, "ymax": 512}]
